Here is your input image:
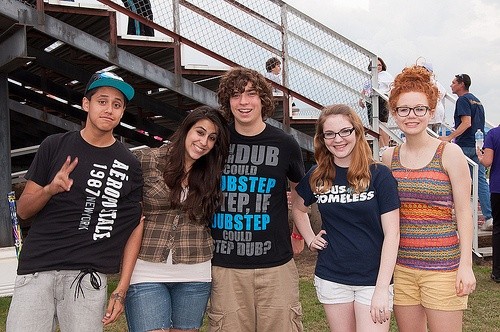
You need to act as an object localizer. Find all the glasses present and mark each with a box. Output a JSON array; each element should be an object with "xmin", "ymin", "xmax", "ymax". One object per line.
[
  {"xmin": 321, "ymin": 127, "xmax": 357, "ymax": 139},
  {"xmin": 459, "ymin": 74, "xmax": 464, "ymax": 78},
  {"xmin": 395, "ymin": 105, "xmax": 432, "ymax": 117}
]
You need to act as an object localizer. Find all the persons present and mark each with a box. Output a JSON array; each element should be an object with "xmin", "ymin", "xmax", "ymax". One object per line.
[
  {"xmin": 359, "ymin": 58, "xmax": 394, "ymax": 162},
  {"xmin": 476, "ymin": 125, "xmax": 500, "ymax": 285},
  {"xmin": 4, "ymin": 72, "xmax": 143, "ymax": 332},
  {"xmin": 209, "ymin": 67, "xmax": 306, "ymax": 332},
  {"xmin": 124, "ymin": 105, "xmax": 231, "ymax": 332},
  {"xmin": 289, "ymin": 105, "xmax": 401, "ymax": 331},
  {"xmin": 380, "ymin": 66, "xmax": 477, "ymax": 332},
  {"xmin": 263, "ymin": 58, "xmax": 296, "ymax": 107},
  {"xmin": 438, "ymin": 73, "xmax": 494, "ymax": 231}
]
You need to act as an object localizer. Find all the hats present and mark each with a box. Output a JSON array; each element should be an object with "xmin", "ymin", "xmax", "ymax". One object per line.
[
  {"xmin": 418, "ymin": 62, "xmax": 433, "ymax": 74},
  {"xmin": 85, "ymin": 71, "xmax": 135, "ymax": 100}
]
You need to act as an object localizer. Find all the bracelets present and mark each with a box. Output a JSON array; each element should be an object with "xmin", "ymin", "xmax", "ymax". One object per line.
[{"xmin": 291, "ymin": 232, "xmax": 304, "ymax": 240}]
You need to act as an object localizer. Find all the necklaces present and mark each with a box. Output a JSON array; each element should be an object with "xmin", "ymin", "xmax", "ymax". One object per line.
[{"xmin": 405, "ymin": 165, "xmax": 412, "ymax": 178}]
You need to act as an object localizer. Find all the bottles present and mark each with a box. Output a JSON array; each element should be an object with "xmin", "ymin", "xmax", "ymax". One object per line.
[{"xmin": 475, "ymin": 129, "xmax": 484, "ymax": 155}]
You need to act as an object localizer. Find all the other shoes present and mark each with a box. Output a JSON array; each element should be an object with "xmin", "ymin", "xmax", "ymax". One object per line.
[
  {"xmin": 481, "ymin": 219, "xmax": 494, "ymax": 230},
  {"xmin": 490, "ymin": 273, "xmax": 500, "ymax": 282}
]
